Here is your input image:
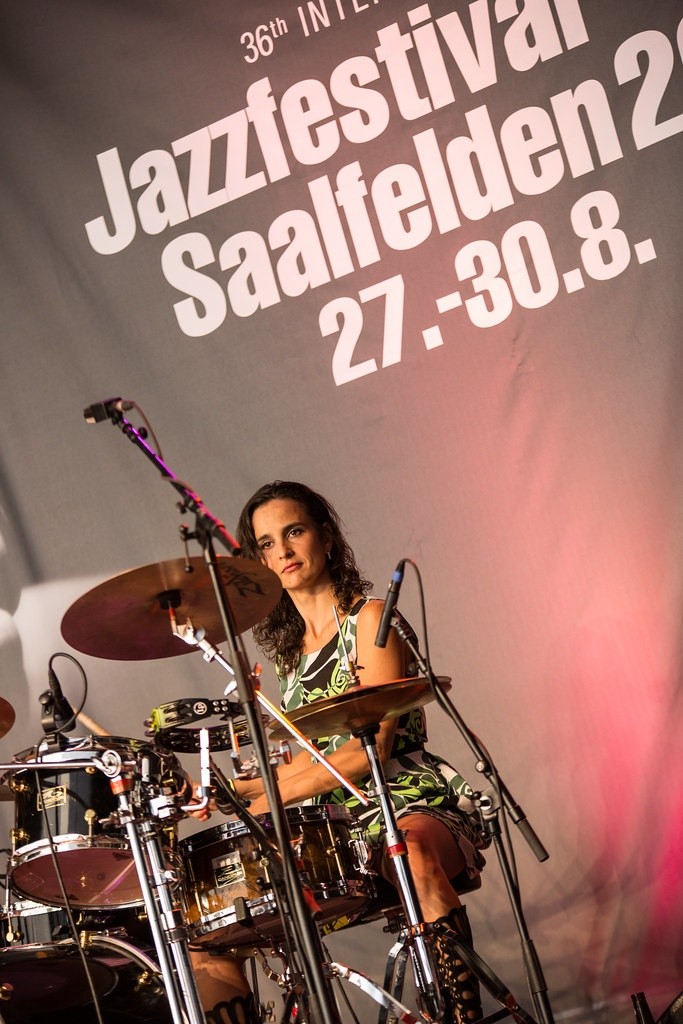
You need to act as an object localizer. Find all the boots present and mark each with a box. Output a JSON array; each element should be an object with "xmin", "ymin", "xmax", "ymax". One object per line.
[
  {"xmin": 416, "ymin": 906, "xmax": 482, "ymax": 1021},
  {"xmin": 198, "ymin": 994, "xmax": 261, "ymax": 1023}
]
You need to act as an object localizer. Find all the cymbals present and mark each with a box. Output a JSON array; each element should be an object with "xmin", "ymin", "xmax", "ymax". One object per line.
[
  {"xmin": 0, "ymin": 698, "xmax": 17, "ymax": 740},
  {"xmin": 268, "ymin": 675, "xmax": 451, "ymax": 742},
  {"xmin": 60, "ymin": 556, "xmax": 283, "ymax": 661},
  {"xmin": 143, "ymin": 697, "xmax": 270, "ymax": 753}
]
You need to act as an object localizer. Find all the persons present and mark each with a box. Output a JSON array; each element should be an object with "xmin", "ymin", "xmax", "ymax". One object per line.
[{"xmin": 148, "ymin": 479, "xmax": 492, "ymax": 1023}]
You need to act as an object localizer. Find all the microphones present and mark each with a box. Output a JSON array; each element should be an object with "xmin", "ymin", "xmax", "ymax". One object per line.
[
  {"xmin": 45, "ymin": 668, "xmax": 77, "ymax": 733},
  {"xmin": 84, "ymin": 398, "xmax": 135, "ymax": 424},
  {"xmin": 375, "ymin": 561, "xmax": 405, "ymax": 648}
]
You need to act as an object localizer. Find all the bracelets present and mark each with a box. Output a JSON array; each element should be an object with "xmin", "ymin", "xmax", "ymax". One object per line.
[{"xmin": 224, "ymin": 778, "xmax": 236, "ymax": 797}]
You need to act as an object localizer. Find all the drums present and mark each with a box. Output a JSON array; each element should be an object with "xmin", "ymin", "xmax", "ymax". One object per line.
[
  {"xmin": 1, "ymin": 942, "xmax": 118, "ymax": 1024},
  {"xmin": 172, "ymin": 804, "xmax": 377, "ymax": 951},
  {"xmin": 50, "ymin": 917, "xmax": 262, "ymax": 1024},
  {"xmin": 7, "ymin": 737, "xmax": 193, "ymax": 912}
]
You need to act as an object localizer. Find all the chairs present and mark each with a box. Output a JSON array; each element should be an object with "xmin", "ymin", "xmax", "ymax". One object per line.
[{"xmin": 212, "ymin": 862, "xmax": 537, "ymax": 1024}]
